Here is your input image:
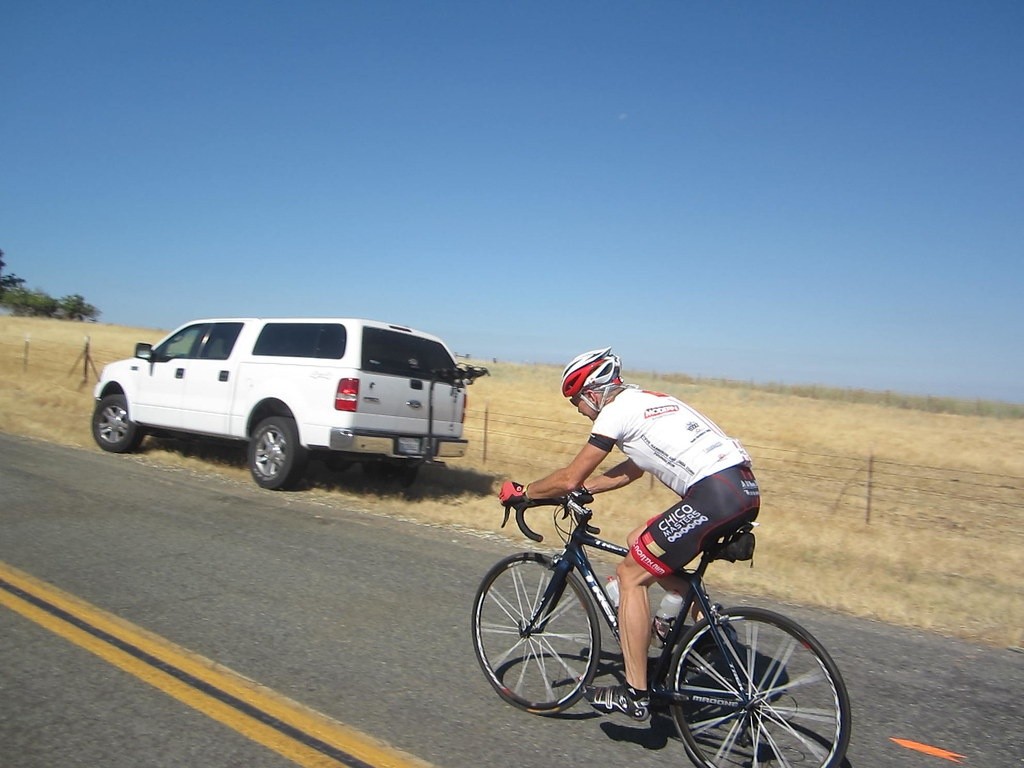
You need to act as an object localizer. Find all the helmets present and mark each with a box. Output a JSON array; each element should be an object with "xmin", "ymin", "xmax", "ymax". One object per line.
[{"xmin": 559, "ymin": 347, "xmax": 623, "ymax": 396}]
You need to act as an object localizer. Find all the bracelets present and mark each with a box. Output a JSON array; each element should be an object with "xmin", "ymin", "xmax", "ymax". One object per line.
[{"xmin": 522, "ymin": 482, "xmax": 533, "ymax": 500}]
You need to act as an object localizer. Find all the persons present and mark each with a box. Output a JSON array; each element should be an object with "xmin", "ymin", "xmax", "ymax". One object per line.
[{"xmin": 492, "ymin": 345, "xmax": 760, "ymax": 721}]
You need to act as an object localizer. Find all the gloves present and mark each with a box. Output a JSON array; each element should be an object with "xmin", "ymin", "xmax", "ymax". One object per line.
[{"xmin": 498, "ymin": 481, "xmax": 530, "ymax": 507}]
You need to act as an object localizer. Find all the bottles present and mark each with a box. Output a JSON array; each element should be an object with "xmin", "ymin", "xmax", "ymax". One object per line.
[
  {"xmin": 650, "ymin": 589, "xmax": 684, "ymax": 648},
  {"xmin": 605, "ymin": 576, "xmax": 619, "ymax": 607}
]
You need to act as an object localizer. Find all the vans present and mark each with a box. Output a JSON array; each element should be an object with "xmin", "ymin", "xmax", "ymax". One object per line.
[{"xmin": 92, "ymin": 318, "xmax": 488, "ymax": 490}]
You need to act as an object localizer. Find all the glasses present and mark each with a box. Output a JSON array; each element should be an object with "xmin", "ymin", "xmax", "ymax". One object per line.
[{"xmin": 569, "ymin": 383, "xmax": 597, "ymax": 407}]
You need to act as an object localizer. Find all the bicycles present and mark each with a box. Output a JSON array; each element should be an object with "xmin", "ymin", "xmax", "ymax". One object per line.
[{"xmin": 470, "ymin": 490, "xmax": 852, "ymax": 768}]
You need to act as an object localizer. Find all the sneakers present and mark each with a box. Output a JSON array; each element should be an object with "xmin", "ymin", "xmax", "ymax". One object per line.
[
  {"xmin": 680, "ymin": 621, "xmax": 739, "ymax": 672},
  {"xmin": 580, "ymin": 684, "xmax": 653, "ymax": 722}
]
